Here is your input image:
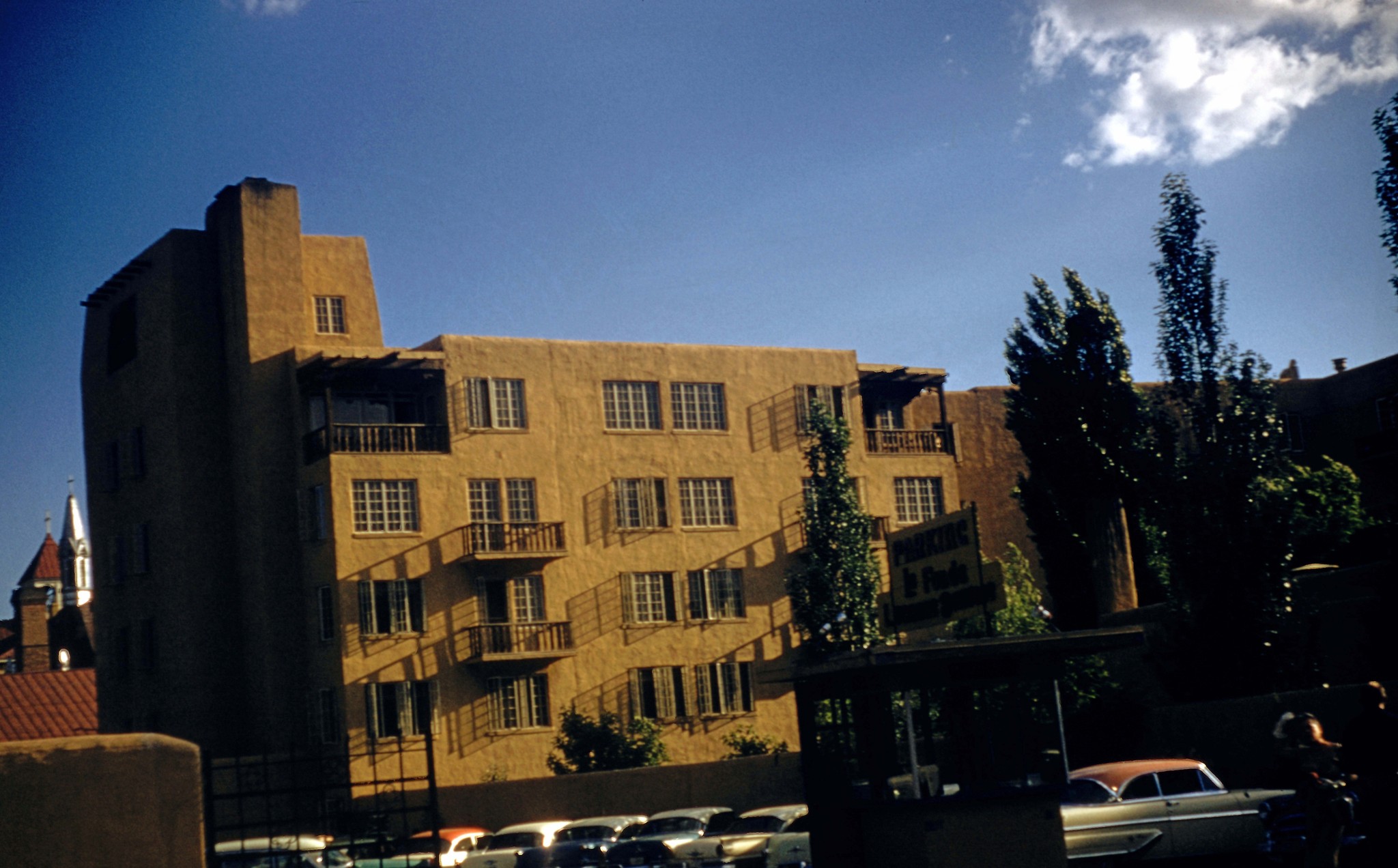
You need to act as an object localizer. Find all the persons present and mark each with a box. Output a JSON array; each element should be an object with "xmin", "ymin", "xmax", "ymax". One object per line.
[{"xmin": 1269, "ymin": 680, "xmax": 1398, "ymax": 868}]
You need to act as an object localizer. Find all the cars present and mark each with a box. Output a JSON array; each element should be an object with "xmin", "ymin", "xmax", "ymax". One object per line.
[
  {"xmin": 1058, "ymin": 759, "xmax": 1298, "ymax": 868},
  {"xmin": 212, "ymin": 803, "xmax": 812, "ymax": 868}
]
